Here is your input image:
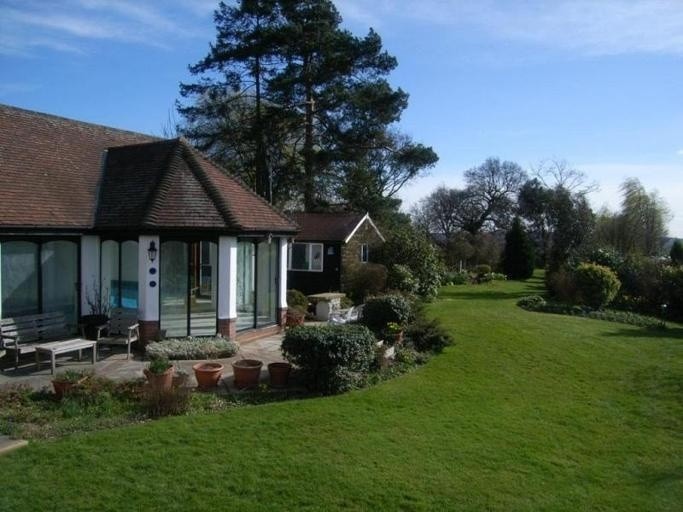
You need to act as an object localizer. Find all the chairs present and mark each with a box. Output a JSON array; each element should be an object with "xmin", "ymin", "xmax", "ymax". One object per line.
[
  {"xmin": 342, "ymin": 303, "xmax": 365, "ymax": 322},
  {"xmin": 326, "ymin": 303, "xmax": 354, "ymax": 326},
  {"xmin": 92, "ymin": 306, "xmax": 142, "ymax": 360}
]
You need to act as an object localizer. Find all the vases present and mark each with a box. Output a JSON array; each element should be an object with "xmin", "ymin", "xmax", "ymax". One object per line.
[
  {"xmin": 267, "ymin": 361, "xmax": 292, "ymax": 386},
  {"xmin": 230, "ymin": 358, "xmax": 263, "ymax": 388},
  {"xmin": 191, "ymin": 359, "xmax": 224, "ymax": 389}
]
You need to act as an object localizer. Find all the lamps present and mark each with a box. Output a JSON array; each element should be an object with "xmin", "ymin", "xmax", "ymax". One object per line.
[{"xmin": 145, "ymin": 240, "xmax": 157, "ymax": 264}]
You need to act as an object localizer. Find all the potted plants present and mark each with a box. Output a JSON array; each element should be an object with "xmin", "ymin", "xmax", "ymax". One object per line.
[
  {"xmin": 142, "ymin": 355, "xmax": 176, "ymax": 392},
  {"xmin": 50, "ymin": 365, "xmax": 84, "ymax": 397}
]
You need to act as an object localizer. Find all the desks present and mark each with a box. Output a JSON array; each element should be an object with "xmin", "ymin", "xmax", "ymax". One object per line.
[
  {"xmin": 34, "ymin": 338, "xmax": 97, "ymax": 375},
  {"xmin": 305, "ymin": 291, "xmax": 347, "ymax": 322}
]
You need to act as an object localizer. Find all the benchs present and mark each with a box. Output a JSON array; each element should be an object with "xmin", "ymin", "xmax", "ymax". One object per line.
[{"xmin": 0, "ymin": 309, "xmax": 88, "ymax": 371}]
